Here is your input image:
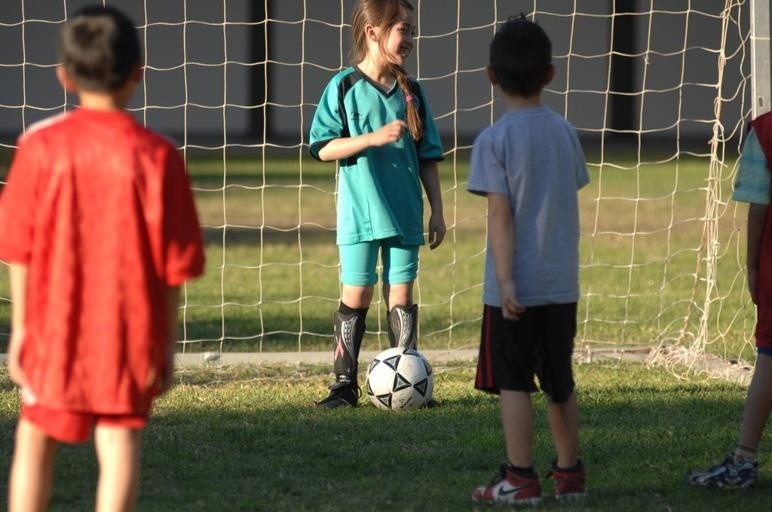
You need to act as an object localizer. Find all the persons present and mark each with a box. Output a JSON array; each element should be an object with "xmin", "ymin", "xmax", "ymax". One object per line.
[
  {"xmin": 464, "ymin": 12, "xmax": 591, "ymax": 505},
  {"xmin": 687, "ymin": 106, "xmax": 772, "ymax": 492},
  {"xmin": 0, "ymin": 5, "xmax": 209, "ymax": 512},
  {"xmin": 306, "ymin": 0, "xmax": 446, "ymax": 409}
]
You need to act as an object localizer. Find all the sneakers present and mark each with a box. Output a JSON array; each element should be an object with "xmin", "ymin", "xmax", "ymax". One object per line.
[
  {"xmin": 689, "ymin": 456, "xmax": 758, "ymax": 488},
  {"xmin": 319, "ymin": 383, "xmax": 362, "ymax": 408},
  {"xmin": 472, "ymin": 462, "xmax": 542, "ymax": 505},
  {"xmin": 546, "ymin": 459, "xmax": 586, "ymax": 499}
]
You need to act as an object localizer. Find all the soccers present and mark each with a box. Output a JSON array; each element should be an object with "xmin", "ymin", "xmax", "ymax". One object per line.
[{"xmin": 367, "ymin": 347, "xmax": 434, "ymax": 411}]
